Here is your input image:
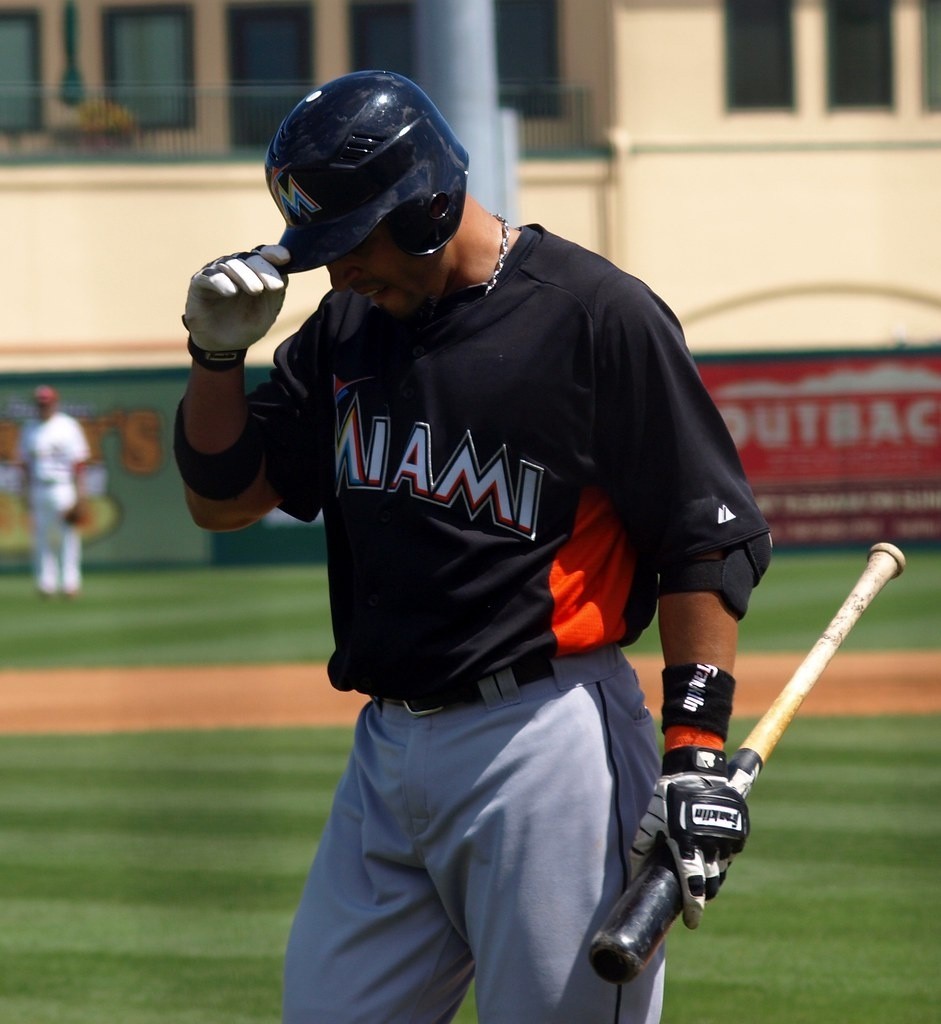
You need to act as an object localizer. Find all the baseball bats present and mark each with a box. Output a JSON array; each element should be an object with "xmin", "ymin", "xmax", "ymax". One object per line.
[{"xmin": 589, "ymin": 542, "xmax": 912, "ymax": 985}]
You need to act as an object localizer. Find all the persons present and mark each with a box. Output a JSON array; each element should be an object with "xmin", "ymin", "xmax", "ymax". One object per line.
[
  {"xmin": 170, "ymin": 69, "xmax": 774, "ymax": 1024},
  {"xmin": 16, "ymin": 386, "xmax": 93, "ymax": 598}
]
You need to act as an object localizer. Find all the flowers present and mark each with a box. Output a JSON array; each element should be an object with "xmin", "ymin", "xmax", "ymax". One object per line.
[{"xmin": 80, "ymin": 95, "xmax": 137, "ymax": 134}]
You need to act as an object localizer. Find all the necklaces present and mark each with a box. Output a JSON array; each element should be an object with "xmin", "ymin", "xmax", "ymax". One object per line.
[{"xmin": 485, "ymin": 214, "xmax": 510, "ymax": 296}]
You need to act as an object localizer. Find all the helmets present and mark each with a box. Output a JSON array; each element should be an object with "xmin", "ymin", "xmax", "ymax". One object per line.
[{"xmin": 265, "ymin": 70, "xmax": 470, "ymax": 275}]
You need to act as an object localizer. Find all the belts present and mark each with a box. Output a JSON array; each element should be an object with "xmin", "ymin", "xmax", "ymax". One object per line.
[{"xmin": 382, "ymin": 658, "xmax": 554, "ymax": 719}]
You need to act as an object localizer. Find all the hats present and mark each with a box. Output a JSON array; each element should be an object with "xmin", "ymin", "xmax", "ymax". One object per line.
[{"xmin": 35, "ymin": 386, "xmax": 56, "ymax": 403}]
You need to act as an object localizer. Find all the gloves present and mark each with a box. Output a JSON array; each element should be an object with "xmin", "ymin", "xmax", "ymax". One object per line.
[
  {"xmin": 628, "ymin": 746, "xmax": 751, "ymax": 930},
  {"xmin": 182, "ymin": 245, "xmax": 291, "ymax": 371}
]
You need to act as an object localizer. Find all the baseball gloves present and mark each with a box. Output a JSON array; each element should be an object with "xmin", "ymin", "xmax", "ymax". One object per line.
[{"xmin": 66, "ymin": 498, "xmax": 92, "ymax": 526}]
